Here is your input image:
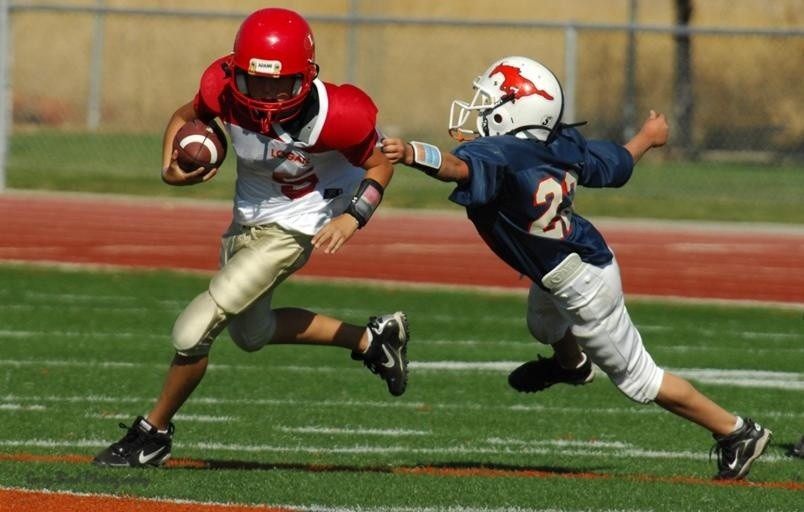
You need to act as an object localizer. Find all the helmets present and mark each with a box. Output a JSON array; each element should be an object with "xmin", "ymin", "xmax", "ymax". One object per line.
[
  {"xmin": 224, "ymin": 9, "xmax": 320, "ymax": 135},
  {"xmin": 447, "ymin": 55, "xmax": 563, "ymax": 144}
]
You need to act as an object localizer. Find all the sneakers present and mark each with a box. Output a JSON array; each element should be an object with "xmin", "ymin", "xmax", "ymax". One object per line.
[
  {"xmin": 92, "ymin": 416, "xmax": 176, "ymax": 467},
  {"xmin": 507, "ymin": 351, "xmax": 595, "ymax": 393},
  {"xmin": 351, "ymin": 310, "xmax": 411, "ymax": 396},
  {"xmin": 708, "ymin": 417, "xmax": 773, "ymax": 481}
]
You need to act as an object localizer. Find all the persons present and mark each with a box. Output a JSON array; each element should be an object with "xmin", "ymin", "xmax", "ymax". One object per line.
[
  {"xmin": 93, "ymin": 5, "xmax": 413, "ymax": 467},
  {"xmin": 377, "ymin": 56, "xmax": 776, "ymax": 486}
]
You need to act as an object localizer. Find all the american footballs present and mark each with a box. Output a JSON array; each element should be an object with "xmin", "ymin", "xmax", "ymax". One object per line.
[{"xmin": 173, "ymin": 119, "xmax": 227, "ymax": 176}]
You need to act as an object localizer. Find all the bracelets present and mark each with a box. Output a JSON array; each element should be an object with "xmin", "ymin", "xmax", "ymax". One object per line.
[
  {"xmin": 342, "ymin": 177, "xmax": 384, "ymax": 230},
  {"xmin": 408, "ymin": 138, "xmax": 444, "ymax": 177}
]
